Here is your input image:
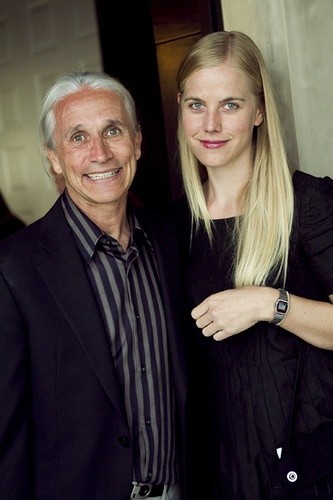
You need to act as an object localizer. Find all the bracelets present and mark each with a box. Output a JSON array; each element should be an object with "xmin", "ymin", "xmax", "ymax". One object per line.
[{"xmin": 275, "ymin": 290, "xmax": 290, "ymax": 326}]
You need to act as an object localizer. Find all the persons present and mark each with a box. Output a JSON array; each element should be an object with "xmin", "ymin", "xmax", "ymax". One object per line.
[
  {"xmin": 0, "ymin": 65, "xmax": 196, "ymax": 500},
  {"xmin": 159, "ymin": 29, "xmax": 333, "ymax": 500}
]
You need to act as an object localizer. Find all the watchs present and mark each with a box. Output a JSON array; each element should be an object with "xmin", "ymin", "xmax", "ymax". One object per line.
[{"xmin": 268, "ymin": 287, "xmax": 288, "ymax": 326}]
